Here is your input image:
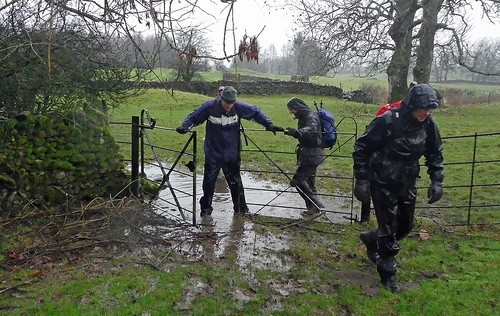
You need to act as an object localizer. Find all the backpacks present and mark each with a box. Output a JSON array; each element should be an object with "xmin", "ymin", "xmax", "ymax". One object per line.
[
  {"xmin": 375, "ymin": 99, "xmax": 401, "ymax": 140},
  {"xmin": 317, "ymin": 106, "xmax": 337, "ymax": 147}
]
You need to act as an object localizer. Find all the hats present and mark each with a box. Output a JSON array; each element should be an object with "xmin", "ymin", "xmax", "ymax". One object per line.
[{"xmin": 221, "ymin": 86, "xmax": 238, "ymax": 102}]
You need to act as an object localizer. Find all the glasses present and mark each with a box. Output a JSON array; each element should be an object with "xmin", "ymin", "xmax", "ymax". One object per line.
[{"xmin": 224, "ymin": 100, "xmax": 235, "ymax": 105}]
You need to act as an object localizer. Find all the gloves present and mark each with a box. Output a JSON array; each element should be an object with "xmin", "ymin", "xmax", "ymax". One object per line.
[
  {"xmin": 427, "ymin": 181, "xmax": 443, "ymax": 204},
  {"xmin": 176, "ymin": 126, "xmax": 189, "ymax": 134},
  {"xmin": 354, "ymin": 180, "xmax": 372, "ymax": 204},
  {"xmin": 266, "ymin": 124, "xmax": 284, "ymax": 135},
  {"xmin": 284, "ymin": 127, "xmax": 302, "ymax": 139}
]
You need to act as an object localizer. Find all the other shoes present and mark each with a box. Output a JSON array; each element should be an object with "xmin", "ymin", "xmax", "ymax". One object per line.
[
  {"xmin": 200, "ymin": 214, "xmax": 213, "ymax": 223},
  {"xmin": 233, "ymin": 212, "xmax": 251, "ymax": 219}
]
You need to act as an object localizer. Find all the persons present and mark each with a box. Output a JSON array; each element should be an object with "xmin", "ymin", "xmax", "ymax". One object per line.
[
  {"xmin": 284, "ymin": 98, "xmax": 324, "ymax": 216},
  {"xmin": 354, "ymin": 84, "xmax": 443, "ymax": 293},
  {"xmin": 176, "ymin": 87, "xmax": 283, "ymax": 222}
]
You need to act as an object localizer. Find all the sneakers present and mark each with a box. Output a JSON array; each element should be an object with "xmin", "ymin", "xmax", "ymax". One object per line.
[
  {"xmin": 359, "ymin": 229, "xmax": 380, "ymax": 264},
  {"xmin": 381, "ymin": 275, "xmax": 403, "ymax": 294}
]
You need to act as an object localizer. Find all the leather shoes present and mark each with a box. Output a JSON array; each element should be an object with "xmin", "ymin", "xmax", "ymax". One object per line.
[{"xmin": 300, "ymin": 202, "xmax": 327, "ymax": 218}]
